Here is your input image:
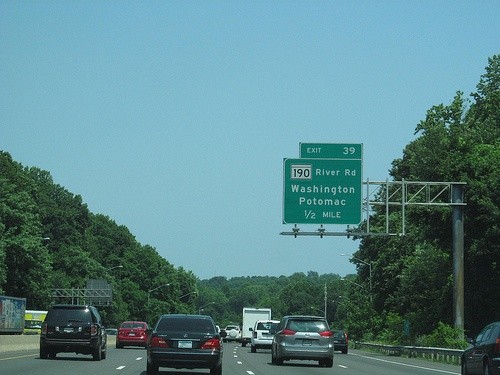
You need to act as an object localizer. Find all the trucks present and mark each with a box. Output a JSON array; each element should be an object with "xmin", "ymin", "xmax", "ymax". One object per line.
[{"xmin": 242, "ymin": 307, "xmax": 272, "ymax": 347}]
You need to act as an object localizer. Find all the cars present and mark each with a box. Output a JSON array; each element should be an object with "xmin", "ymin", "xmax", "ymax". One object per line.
[
  {"xmin": 331, "ymin": 330, "xmax": 349, "ymax": 355},
  {"xmin": 215, "ymin": 324, "xmax": 242, "ymax": 344},
  {"xmin": 116, "ymin": 320, "xmax": 150, "ymax": 349},
  {"xmin": 271, "ymin": 316, "xmax": 334, "ymax": 367},
  {"xmin": 146, "ymin": 314, "xmax": 225, "ymax": 375},
  {"xmin": 458, "ymin": 321, "xmax": 500, "ymax": 375}
]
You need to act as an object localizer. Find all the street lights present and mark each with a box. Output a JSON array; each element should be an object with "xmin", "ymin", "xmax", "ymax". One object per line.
[
  {"xmin": 199, "ymin": 301, "xmax": 216, "ymax": 316},
  {"xmin": 341, "ymin": 253, "xmax": 375, "ymax": 303},
  {"xmin": 309, "ymin": 284, "xmax": 328, "ymax": 316},
  {"xmin": 147, "ymin": 283, "xmax": 171, "ymax": 323}
]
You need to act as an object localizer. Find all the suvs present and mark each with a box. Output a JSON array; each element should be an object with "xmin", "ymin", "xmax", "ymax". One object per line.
[
  {"xmin": 41, "ymin": 304, "xmax": 108, "ymax": 360},
  {"xmin": 249, "ymin": 320, "xmax": 281, "ymax": 353}
]
